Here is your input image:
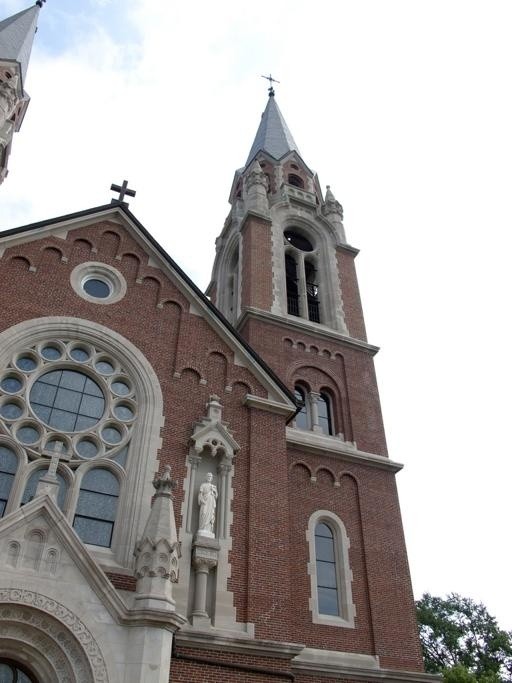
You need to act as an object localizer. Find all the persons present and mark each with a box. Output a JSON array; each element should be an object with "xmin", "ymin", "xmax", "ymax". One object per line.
[{"xmin": 198, "ymin": 472, "xmax": 218, "ymax": 532}]
